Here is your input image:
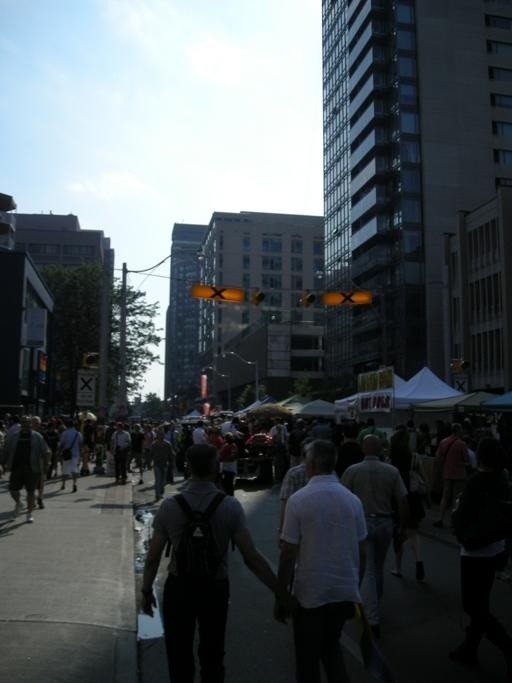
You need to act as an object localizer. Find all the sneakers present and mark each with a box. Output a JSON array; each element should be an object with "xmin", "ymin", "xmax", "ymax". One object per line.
[{"xmin": 12, "ymin": 502, "xmax": 44, "ymax": 523}]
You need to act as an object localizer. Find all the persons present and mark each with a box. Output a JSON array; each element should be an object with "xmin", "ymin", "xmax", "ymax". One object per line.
[
  {"xmin": 342, "ymin": 436, "xmax": 408, "ymax": 625},
  {"xmin": 277, "ymin": 437, "xmax": 369, "ymax": 683},
  {"xmin": 447, "ymin": 438, "xmax": 512, "ymax": 667},
  {"xmin": 141, "ymin": 443, "xmax": 294, "ymax": 683}
]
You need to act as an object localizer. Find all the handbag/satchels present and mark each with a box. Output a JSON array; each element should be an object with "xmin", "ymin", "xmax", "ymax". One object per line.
[
  {"xmin": 451, "ymin": 492, "xmax": 510, "ymax": 552},
  {"xmin": 62, "ymin": 448, "xmax": 72, "ymax": 461},
  {"xmin": 431, "ymin": 455, "xmax": 445, "ymax": 477},
  {"xmin": 395, "ymin": 490, "xmax": 426, "ymax": 528},
  {"xmin": 114, "ymin": 448, "xmax": 129, "ymax": 462}
]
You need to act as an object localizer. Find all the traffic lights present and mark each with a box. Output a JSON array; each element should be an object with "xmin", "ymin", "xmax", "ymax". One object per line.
[
  {"xmin": 255, "ymin": 292, "xmax": 265, "ymax": 301},
  {"xmin": 306, "ymin": 294, "xmax": 316, "ymax": 303}
]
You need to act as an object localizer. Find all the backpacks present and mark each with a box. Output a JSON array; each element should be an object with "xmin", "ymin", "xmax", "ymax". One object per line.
[{"xmin": 172, "ymin": 490, "xmax": 230, "ymax": 620}]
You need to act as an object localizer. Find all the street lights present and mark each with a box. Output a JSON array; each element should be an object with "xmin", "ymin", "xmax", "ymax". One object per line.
[
  {"xmin": 119, "ymin": 245, "xmax": 208, "ymax": 403},
  {"xmin": 200, "ymin": 351, "xmax": 260, "ymax": 403},
  {"xmin": 82, "ymin": 352, "xmax": 97, "ymax": 368}
]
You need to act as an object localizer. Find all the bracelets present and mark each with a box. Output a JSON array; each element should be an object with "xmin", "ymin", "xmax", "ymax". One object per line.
[{"xmin": 142, "ymin": 588, "xmax": 154, "ymax": 593}]
